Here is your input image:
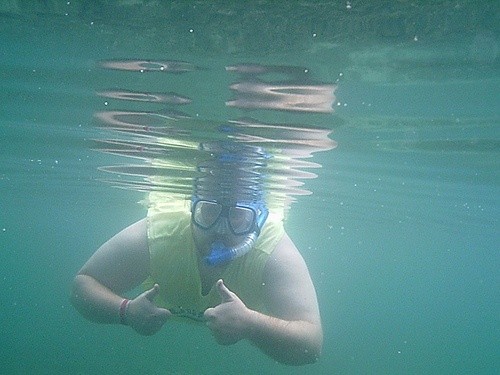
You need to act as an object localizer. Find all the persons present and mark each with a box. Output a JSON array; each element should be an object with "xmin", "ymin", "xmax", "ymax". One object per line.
[{"xmin": 70, "ymin": 198, "xmax": 322, "ymax": 366}]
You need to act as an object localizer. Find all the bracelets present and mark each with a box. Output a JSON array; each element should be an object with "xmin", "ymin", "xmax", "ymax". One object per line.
[{"xmin": 119, "ymin": 299, "xmax": 131, "ymax": 326}]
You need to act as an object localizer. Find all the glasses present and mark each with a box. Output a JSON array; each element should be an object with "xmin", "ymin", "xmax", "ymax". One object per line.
[{"xmin": 189, "ymin": 199, "xmax": 258, "ymax": 237}]
